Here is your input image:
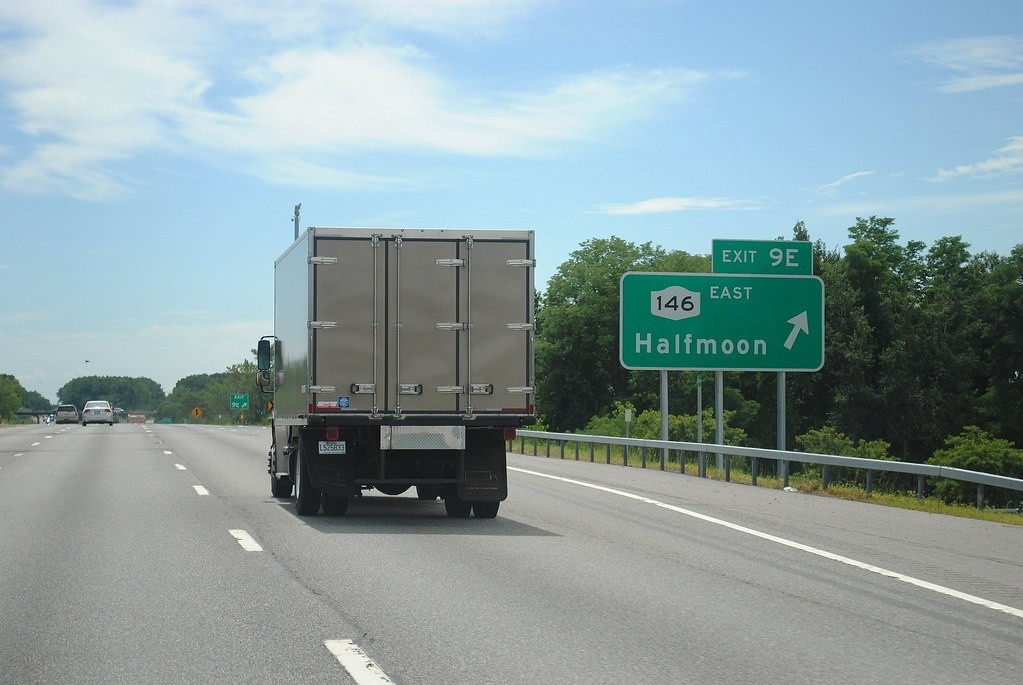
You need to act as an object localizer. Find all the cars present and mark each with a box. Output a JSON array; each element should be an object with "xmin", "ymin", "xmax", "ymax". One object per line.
[
  {"xmin": 110, "ymin": 402, "xmax": 120, "ymax": 423},
  {"xmin": 81, "ymin": 400, "xmax": 114, "ymax": 426},
  {"xmin": 56, "ymin": 404, "xmax": 78, "ymax": 424},
  {"xmin": 127, "ymin": 413, "xmax": 146, "ymax": 423},
  {"xmin": 42, "ymin": 413, "xmax": 55, "ymax": 423}
]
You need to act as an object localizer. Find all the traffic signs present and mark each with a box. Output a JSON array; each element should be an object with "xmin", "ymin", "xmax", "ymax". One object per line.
[
  {"xmin": 230, "ymin": 393, "xmax": 250, "ymax": 411},
  {"xmin": 619, "ymin": 270, "xmax": 826, "ymax": 372}
]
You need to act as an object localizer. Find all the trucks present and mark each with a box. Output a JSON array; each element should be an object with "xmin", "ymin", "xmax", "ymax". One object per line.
[{"xmin": 257, "ymin": 225, "xmax": 537, "ymax": 519}]
